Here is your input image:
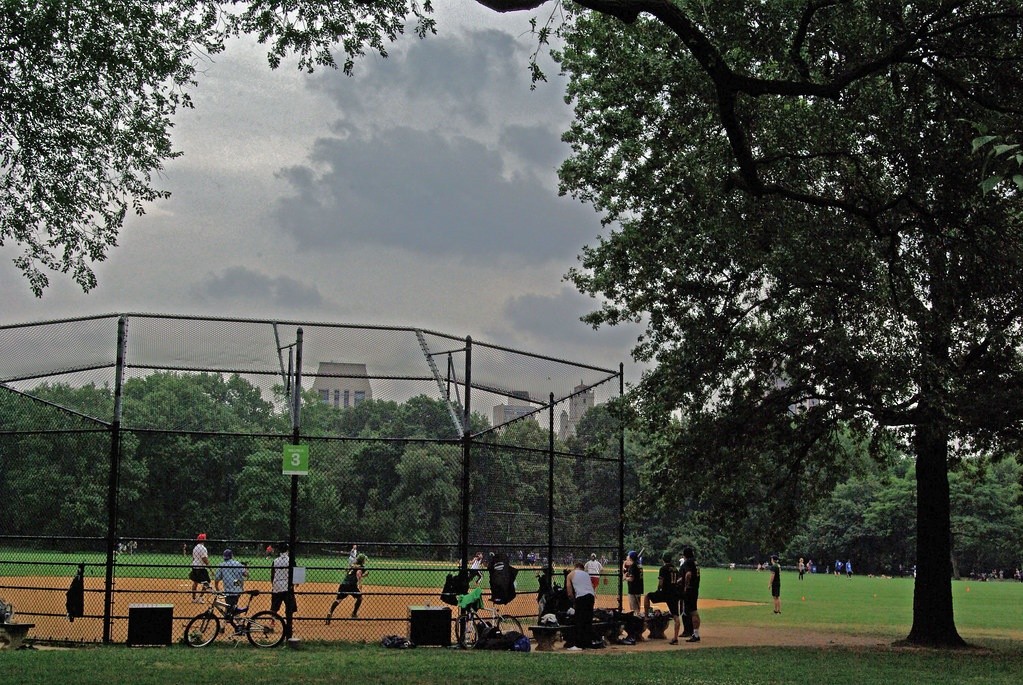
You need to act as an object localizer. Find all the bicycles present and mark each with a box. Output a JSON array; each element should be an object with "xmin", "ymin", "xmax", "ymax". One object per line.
[
  {"xmin": 455, "ymin": 587, "xmax": 523, "ymax": 650},
  {"xmin": 184, "ymin": 586, "xmax": 286, "ymax": 648}
]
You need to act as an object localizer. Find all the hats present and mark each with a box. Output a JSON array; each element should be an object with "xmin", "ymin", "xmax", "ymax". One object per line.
[
  {"xmin": 591, "ymin": 553, "xmax": 596, "ymax": 558},
  {"xmin": 223, "ymin": 549, "xmax": 233, "ymax": 559},
  {"xmin": 771, "ymin": 555, "xmax": 779, "ymax": 563},
  {"xmin": 198, "ymin": 534, "xmax": 206, "ymax": 540},
  {"xmin": 627, "ymin": 550, "xmax": 637, "ymax": 556}
]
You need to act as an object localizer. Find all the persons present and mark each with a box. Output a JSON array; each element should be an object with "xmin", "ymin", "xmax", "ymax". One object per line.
[
  {"xmin": 642, "ymin": 547, "xmax": 700, "ymax": 645},
  {"xmin": 756, "ymin": 562, "xmax": 770, "ymax": 571},
  {"xmin": 622, "ymin": 550, "xmax": 644, "ymax": 614},
  {"xmin": 833, "ymin": 559, "xmax": 853, "ymax": 580},
  {"xmin": 269, "ymin": 542, "xmax": 297, "ymax": 628},
  {"xmin": 349, "ymin": 545, "xmax": 357, "ymax": 566},
  {"xmin": 213, "ymin": 549, "xmax": 254, "ymax": 614},
  {"xmin": 113, "ymin": 539, "xmax": 137, "ymax": 555},
  {"xmin": 325, "ymin": 545, "xmax": 369, "ymax": 625},
  {"xmin": 265, "ymin": 545, "xmax": 274, "ymax": 559},
  {"xmin": 583, "ymin": 553, "xmax": 604, "ymax": 588},
  {"xmin": 799, "ymin": 558, "xmax": 814, "ymax": 580},
  {"xmin": 767, "ymin": 554, "xmax": 781, "ymax": 614},
  {"xmin": 188, "ymin": 533, "xmax": 215, "ymax": 604},
  {"xmin": 725, "ymin": 561, "xmax": 737, "ymax": 569},
  {"xmin": 867, "ymin": 564, "xmax": 916, "ymax": 579},
  {"xmin": 566, "ymin": 561, "xmax": 594, "ymax": 650},
  {"xmin": 967, "ymin": 568, "xmax": 1020, "ymax": 581},
  {"xmin": 468, "ymin": 550, "xmax": 608, "ymax": 568}
]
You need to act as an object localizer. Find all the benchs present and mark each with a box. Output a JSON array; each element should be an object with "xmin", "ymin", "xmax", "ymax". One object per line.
[
  {"xmin": 0, "ymin": 623, "xmax": 34, "ymax": 649},
  {"xmin": 527, "ymin": 617, "xmax": 646, "ymax": 650}
]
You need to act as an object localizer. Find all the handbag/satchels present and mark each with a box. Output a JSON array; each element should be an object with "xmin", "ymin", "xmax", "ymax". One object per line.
[{"xmin": 511, "ymin": 636, "xmax": 531, "ymax": 652}]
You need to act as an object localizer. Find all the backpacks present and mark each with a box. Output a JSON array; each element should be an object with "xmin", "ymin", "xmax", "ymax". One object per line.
[
  {"xmin": 483, "ymin": 626, "xmax": 503, "ymax": 639},
  {"xmin": 488, "ymin": 553, "xmax": 519, "ymax": 606},
  {"xmin": 476, "ymin": 621, "xmax": 492, "ymax": 639},
  {"xmin": 440, "ymin": 573, "xmax": 470, "ymax": 607}
]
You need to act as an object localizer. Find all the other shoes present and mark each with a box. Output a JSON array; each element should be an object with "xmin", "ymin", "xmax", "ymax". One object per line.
[
  {"xmin": 219, "ymin": 628, "xmax": 224, "ymax": 633},
  {"xmin": 192, "ymin": 596, "xmax": 205, "ymax": 604},
  {"xmin": 270, "ymin": 619, "xmax": 274, "ymax": 627},
  {"xmin": 644, "ymin": 616, "xmax": 649, "ymax": 622},
  {"xmin": 686, "ymin": 635, "xmax": 700, "ymax": 643},
  {"xmin": 669, "ymin": 639, "xmax": 678, "ymax": 645},
  {"xmin": 621, "ymin": 636, "xmax": 635, "ymax": 645},
  {"xmin": 348, "ymin": 612, "xmax": 360, "ymax": 618},
  {"xmin": 774, "ymin": 610, "xmax": 782, "ymax": 615},
  {"xmin": 678, "ymin": 632, "xmax": 694, "ymax": 636},
  {"xmin": 326, "ymin": 612, "xmax": 332, "ymax": 625},
  {"xmin": 567, "ymin": 646, "xmax": 582, "ymax": 651}
]
